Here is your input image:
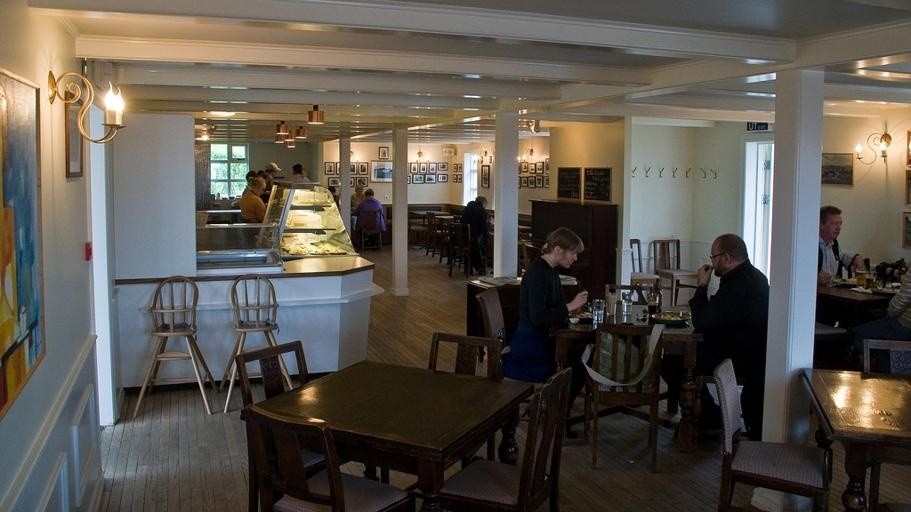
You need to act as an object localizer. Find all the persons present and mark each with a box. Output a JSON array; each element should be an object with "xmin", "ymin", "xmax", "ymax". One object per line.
[
  {"xmin": 353, "ymin": 188, "xmax": 388, "ymax": 253},
  {"xmin": 350, "ymin": 184, "xmax": 369, "ymax": 208},
  {"xmin": 819, "ymin": 204, "xmax": 868, "ymax": 369},
  {"xmin": 328, "ymin": 186, "xmax": 337, "ymax": 203},
  {"xmin": 502, "ymin": 225, "xmax": 592, "ymax": 444},
  {"xmin": 238, "ymin": 162, "xmax": 311, "ymax": 222},
  {"xmin": 663, "ymin": 233, "xmax": 769, "ymax": 433},
  {"xmin": 457, "ymin": 196, "xmax": 493, "ymax": 275},
  {"xmin": 833, "ymin": 260, "xmax": 910, "ymax": 378}
]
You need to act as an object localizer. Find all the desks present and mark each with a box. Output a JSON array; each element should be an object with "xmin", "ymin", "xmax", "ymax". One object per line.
[{"xmin": 241, "ymin": 360, "xmax": 535, "ymax": 512}]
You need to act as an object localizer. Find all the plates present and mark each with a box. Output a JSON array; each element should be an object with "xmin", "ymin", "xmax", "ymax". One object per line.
[
  {"xmin": 873, "ymin": 288, "xmax": 899, "ymax": 295},
  {"xmin": 649, "ymin": 310, "xmax": 691, "ymax": 324}
]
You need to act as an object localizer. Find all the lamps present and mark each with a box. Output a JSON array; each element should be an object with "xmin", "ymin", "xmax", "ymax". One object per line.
[
  {"xmin": 48, "ymin": 70, "xmax": 128, "ymax": 143},
  {"xmin": 275, "ymin": 103, "xmax": 323, "ymax": 150},
  {"xmin": 851, "ymin": 131, "xmax": 895, "ymax": 172}
]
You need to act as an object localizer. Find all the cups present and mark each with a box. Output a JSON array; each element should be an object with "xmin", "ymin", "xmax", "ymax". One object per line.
[
  {"xmin": 590, "ymin": 300, "xmax": 604, "ymax": 323},
  {"xmin": 605, "ymin": 283, "xmax": 632, "ymax": 317},
  {"xmin": 855, "ymin": 267, "xmax": 877, "ymax": 288}
]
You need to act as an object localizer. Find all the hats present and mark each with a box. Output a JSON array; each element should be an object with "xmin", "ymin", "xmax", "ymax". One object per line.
[{"xmin": 266, "ymin": 162, "xmax": 281, "ymax": 171}]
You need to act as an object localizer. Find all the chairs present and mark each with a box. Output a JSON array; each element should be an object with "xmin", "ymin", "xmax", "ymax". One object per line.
[
  {"xmin": 435, "ymin": 367, "xmax": 574, "ymax": 512},
  {"xmin": 234, "ymin": 339, "xmax": 353, "ymax": 512},
  {"xmin": 220, "ymin": 272, "xmax": 295, "ymax": 412},
  {"xmin": 132, "ymin": 276, "xmax": 219, "ymax": 418},
  {"xmin": 379, "ymin": 333, "xmax": 507, "ymax": 512},
  {"xmin": 245, "ymin": 403, "xmax": 409, "ymax": 512},
  {"xmin": 354, "ymin": 205, "xmax": 535, "ymax": 277}
]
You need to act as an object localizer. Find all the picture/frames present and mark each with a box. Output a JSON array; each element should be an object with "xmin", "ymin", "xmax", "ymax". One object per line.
[
  {"xmin": 901, "ymin": 128, "xmax": 911, "ymax": 251},
  {"xmin": 820, "ymin": 152, "xmax": 854, "ymax": 188},
  {"xmin": 63, "ymin": 91, "xmax": 86, "ymax": 178},
  {"xmin": 323, "ymin": 145, "xmax": 551, "ymax": 190},
  {"xmin": 0, "ymin": 66, "xmax": 47, "ymax": 426}
]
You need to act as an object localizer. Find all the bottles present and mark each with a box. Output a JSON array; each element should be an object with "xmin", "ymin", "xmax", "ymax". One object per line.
[{"xmin": 645, "ymin": 279, "xmax": 663, "ymax": 313}]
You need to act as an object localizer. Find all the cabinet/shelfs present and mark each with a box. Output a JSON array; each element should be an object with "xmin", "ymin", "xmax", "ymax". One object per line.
[{"xmin": 260, "ymin": 181, "xmax": 355, "ymax": 260}]
